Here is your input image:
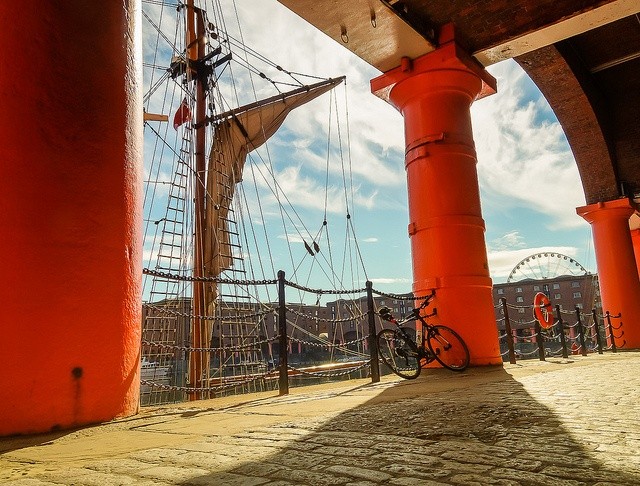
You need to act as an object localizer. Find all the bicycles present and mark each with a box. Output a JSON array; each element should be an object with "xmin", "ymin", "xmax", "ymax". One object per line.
[{"xmin": 368, "ymin": 289, "xmax": 469, "ymax": 380}]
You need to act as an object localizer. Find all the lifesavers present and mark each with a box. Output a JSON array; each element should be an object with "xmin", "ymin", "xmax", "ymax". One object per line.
[{"xmin": 534, "ymin": 292, "xmax": 554, "ymax": 329}]
